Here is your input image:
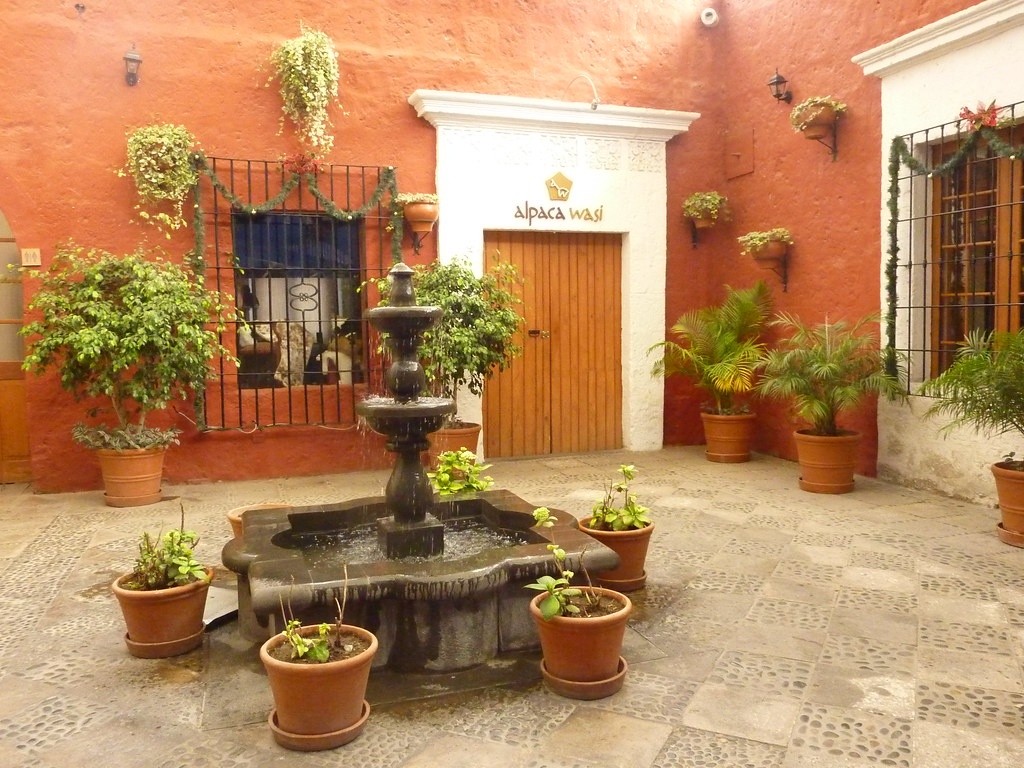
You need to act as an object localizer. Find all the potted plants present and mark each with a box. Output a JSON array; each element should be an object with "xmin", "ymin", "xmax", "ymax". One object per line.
[
  {"xmin": 580, "ymin": 464, "xmax": 657, "ymax": 597},
  {"xmin": 123, "ymin": 124, "xmax": 201, "ymax": 229},
  {"xmin": 400, "ymin": 187, "xmax": 443, "ymax": 233},
  {"xmin": 766, "ymin": 312, "xmax": 908, "ymax": 492},
  {"xmin": 387, "ymin": 254, "xmax": 521, "ymax": 473},
  {"xmin": 10, "ymin": 248, "xmax": 246, "ymax": 507},
  {"xmin": 923, "ymin": 327, "xmax": 1024, "ymax": 548},
  {"xmin": 257, "ymin": 559, "xmax": 380, "ymax": 752},
  {"xmin": 653, "ymin": 282, "xmax": 774, "ymax": 461},
  {"xmin": 790, "ymin": 95, "xmax": 845, "ymax": 141},
  {"xmin": 683, "ymin": 188, "xmax": 734, "ymax": 227},
  {"xmin": 111, "ymin": 502, "xmax": 216, "ymax": 658},
  {"xmin": 529, "ymin": 505, "xmax": 635, "ymax": 703},
  {"xmin": 738, "ymin": 226, "xmax": 791, "ymax": 265}
]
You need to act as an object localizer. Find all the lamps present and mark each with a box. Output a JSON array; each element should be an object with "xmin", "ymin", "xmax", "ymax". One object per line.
[
  {"xmin": 123, "ymin": 37, "xmax": 143, "ymax": 84},
  {"xmin": 769, "ymin": 67, "xmax": 794, "ymax": 106},
  {"xmin": 564, "ymin": 74, "xmax": 600, "ymax": 110}
]
